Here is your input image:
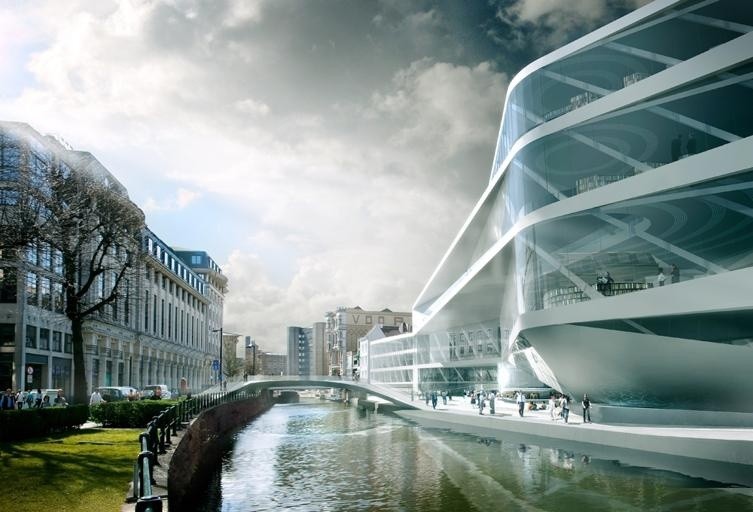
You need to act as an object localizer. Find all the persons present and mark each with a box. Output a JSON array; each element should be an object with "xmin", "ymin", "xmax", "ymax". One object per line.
[
  {"xmin": 549, "ymin": 393, "xmax": 570, "ymax": 424},
  {"xmin": 128, "ymin": 387, "xmax": 161, "ymax": 401},
  {"xmin": 517, "ymin": 392, "xmax": 526, "ymax": 417},
  {"xmin": 418, "ymin": 390, "xmax": 452, "ymax": 410},
  {"xmin": 464, "ymin": 389, "xmax": 496, "ymax": 415},
  {"xmin": 582, "ymin": 394, "xmax": 592, "ymax": 423},
  {"xmin": 670, "ymin": 264, "xmax": 679, "ymax": 283},
  {"xmin": 89, "ymin": 389, "xmax": 107, "ymax": 405},
  {"xmin": 596, "ymin": 271, "xmax": 615, "ymax": 297},
  {"xmin": 0, "ymin": 389, "xmax": 69, "ymax": 410},
  {"xmin": 528, "ymin": 401, "xmax": 546, "ymax": 410},
  {"xmin": 657, "ymin": 271, "xmax": 667, "ymax": 286}
]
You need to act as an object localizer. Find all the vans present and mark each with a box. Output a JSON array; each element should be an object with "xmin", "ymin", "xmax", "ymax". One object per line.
[{"xmin": 98, "ymin": 385, "xmax": 167, "ymax": 402}]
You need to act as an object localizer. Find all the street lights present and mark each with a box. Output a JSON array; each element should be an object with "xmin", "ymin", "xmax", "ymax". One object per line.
[
  {"xmin": 246, "ymin": 344, "xmax": 256, "ymax": 374},
  {"xmin": 212, "ymin": 328, "xmax": 223, "ymax": 391}
]
